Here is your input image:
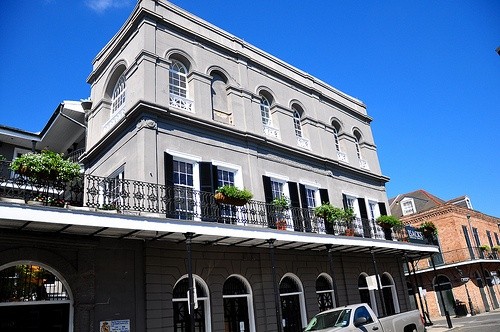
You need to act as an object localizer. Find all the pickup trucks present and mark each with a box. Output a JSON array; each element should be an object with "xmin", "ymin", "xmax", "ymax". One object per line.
[{"xmin": 303, "ymin": 303, "xmax": 426, "ymax": 332}]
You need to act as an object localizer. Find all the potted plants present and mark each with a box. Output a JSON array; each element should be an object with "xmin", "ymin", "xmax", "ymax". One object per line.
[
  {"xmin": 314, "ymin": 202, "xmax": 345, "ymax": 223},
  {"xmin": 215, "ymin": 185, "xmax": 253, "ymax": 206},
  {"xmin": 10, "ymin": 149, "xmax": 79, "ymax": 180},
  {"xmin": 272, "ymin": 193, "xmax": 289, "ymax": 230},
  {"xmin": 376, "ymin": 215, "xmax": 404, "ymax": 231},
  {"xmin": 341, "ymin": 209, "xmax": 356, "ymax": 236},
  {"xmin": 420, "ymin": 222, "xmax": 438, "ymax": 237}
]
